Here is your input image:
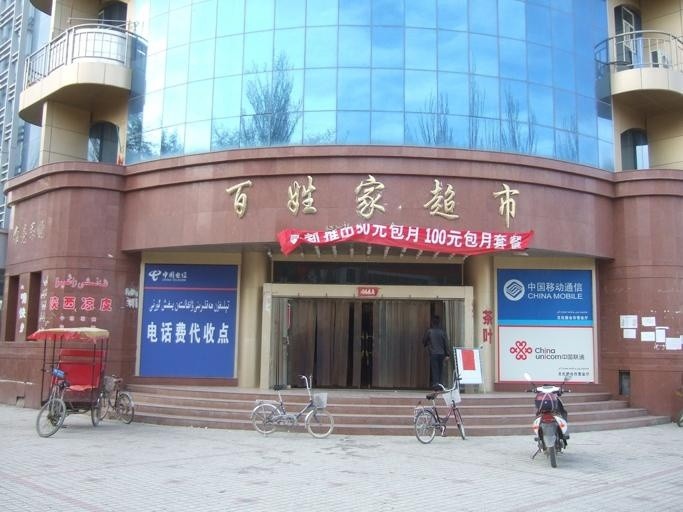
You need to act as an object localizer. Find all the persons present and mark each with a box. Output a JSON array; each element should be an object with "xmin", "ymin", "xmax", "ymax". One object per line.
[{"xmin": 422, "ymin": 316, "xmax": 450, "ymax": 389}]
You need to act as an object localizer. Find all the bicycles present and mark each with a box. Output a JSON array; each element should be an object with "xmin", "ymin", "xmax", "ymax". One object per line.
[
  {"xmin": 249, "ymin": 373, "xmax": 334, "ymax": 438},
  {"xmin": 414, "ymin": 372, "xmax": 466, "ymax": 444},
  {"xmin": 96, "ymin": 374, "xmax": 134, "ymax": 424}
]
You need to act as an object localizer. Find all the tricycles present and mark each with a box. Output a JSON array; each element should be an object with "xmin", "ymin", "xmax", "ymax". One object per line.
[{"xmin": 26, "ymin": 327, "xmax": 109, "ymax": 438}]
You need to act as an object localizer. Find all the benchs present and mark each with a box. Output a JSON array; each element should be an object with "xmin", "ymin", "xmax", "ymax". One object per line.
[{"xmin": 51, "ymin": 360, "xmax": 102, "ymax": 392}]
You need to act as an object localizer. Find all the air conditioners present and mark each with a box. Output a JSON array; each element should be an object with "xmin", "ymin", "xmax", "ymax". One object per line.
[{"xmin": 649, "ymin": 47, "xmax": 672, "ymax": 70}]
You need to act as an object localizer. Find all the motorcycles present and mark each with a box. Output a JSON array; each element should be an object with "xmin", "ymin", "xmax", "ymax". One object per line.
[{"xmin": 522, "ymin": 372, "xmax": 572, "ymax": 467}]
